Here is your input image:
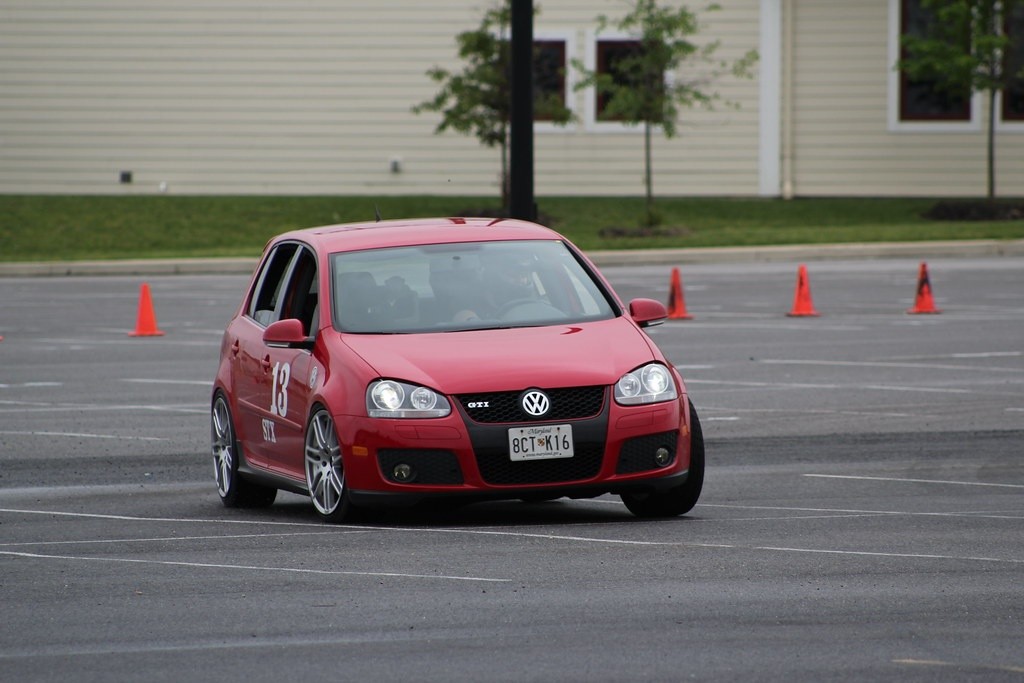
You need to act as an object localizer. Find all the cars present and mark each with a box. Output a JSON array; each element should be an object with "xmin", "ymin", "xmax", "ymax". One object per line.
[{"xmin": 210, "ymin": 214, "xmax": 705, "ymax": 526}]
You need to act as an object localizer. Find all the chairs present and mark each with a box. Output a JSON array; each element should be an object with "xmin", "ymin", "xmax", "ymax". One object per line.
[
  {"xmin": 423, "ymin": 263, "xmax": 480, "ymax": 324},
  {"xmin": 338, "ymin": 272, "xmax": 378, "ymax": 322}
]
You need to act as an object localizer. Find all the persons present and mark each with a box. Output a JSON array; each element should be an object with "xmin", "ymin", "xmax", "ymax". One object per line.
[{"xmin": 451, "ymin": 257, "xmax": 540, "ymax": 324}]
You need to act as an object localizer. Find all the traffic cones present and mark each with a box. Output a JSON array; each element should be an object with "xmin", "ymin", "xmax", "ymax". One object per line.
[
  {"xmin": 127, "ymin": 281, "xmax": 165, "ymax": 336},
  {"xmin": 784, "ymin": 262, "xmax": 823, "ymax": 316},
  {"xmin": 663, "ymin": 266, "xmax": 696, "ymax": 320},
  {"xmin": 908, "ymin": 261, "xmax": 942, "ymax": 316}
]
